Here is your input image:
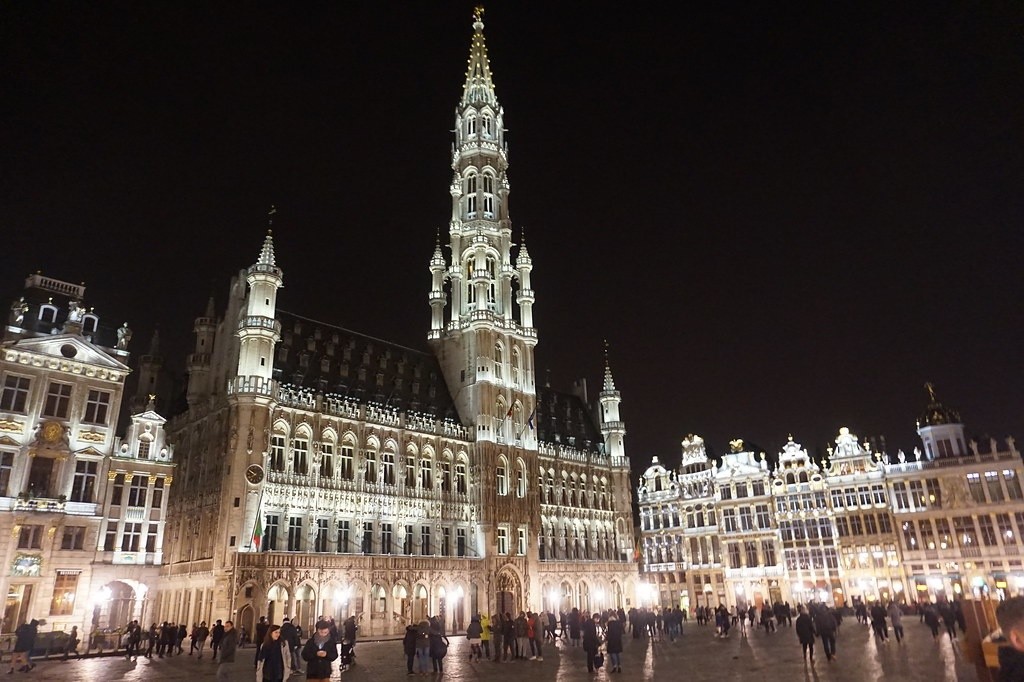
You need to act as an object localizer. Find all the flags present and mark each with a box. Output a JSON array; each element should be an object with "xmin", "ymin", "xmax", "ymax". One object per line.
[
  {"xmin": 529, "ymin": 409, "xmax": 536, "ymax": 430},
  {"xmin": 253, "ymin": 515, "xmax": 263, "ymax": 549},
  {"xmin": 508, "ymin": 404, "xmax": 514, "ymax": 417},
  {"xmin": 634, "ymin": 544, "xmax": 644, "ymax": 559}
]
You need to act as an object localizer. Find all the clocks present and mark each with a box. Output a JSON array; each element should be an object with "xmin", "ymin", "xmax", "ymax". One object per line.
[{"xmin": 245, "ymin": 464, "xmax": 265, "ymax": 485}]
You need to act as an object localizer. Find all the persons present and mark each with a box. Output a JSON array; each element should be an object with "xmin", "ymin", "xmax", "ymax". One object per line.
[
  {"xmin": 123, "ymin": 619, "xmax": 246, "ymax": 660},
  {"xmin": 995, "ymin": 595, "xmax": 1024, "ymax": 682},
  {"xmin": 851, "ymin": 595, "xmax": 903, "ymax": 643},
  {"xmin": 466, "ymin": 608, "xmax": 591, "ymax": 664},
  {"xmin": 967, "ymin": 435, "xmax": 1015, "ymax": 457},
  {"xmin": 693, "ymin": 598, "xmax": 843, "ymax": 662},
  {"xmin": 10, "ymin": 296, "xmax": 29, "ymax": 326},
  {"xmin": 6, "ymin": 619, "xmax": 39, "ymax": 673},
  {"xmin": 882, "ymin": 446, "xmax": 921, "ymax": 464},
  {"xmin": 217, "ymin": 620, "xmax": 237, "ymax": 682},
  {"xmin": 60, "ymin": 625, "xmax": 81, "ymax": 661},
  {"xmin": 117, "ymin": 321, "xmax": 133, "ymax": 349},
  {"xmin": 913, "ymin": 596, "xmax": 967, "ymax": 642},
  {"xmin": 254, "ymin": 615, "xmax": 357, "ymax": 682},
  {"xmin": 628, "ymin": 605, "xmax": 687, "ymax": 642},
  {"xmin": 402, "ymin": 614, "xmax": 450, "ymax": 675},
  {"xmin": 582, "ymin": 606, "xmax": 626, "ymax": 673}
]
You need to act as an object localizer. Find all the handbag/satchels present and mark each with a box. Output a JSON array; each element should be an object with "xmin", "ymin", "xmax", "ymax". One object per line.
[
  {"xmin": 121, "ymin": 631, "xmax": 131, "ymax": 646},
  {"xmin": 716, "ymin": 624, "xmax": 721, "ymax": 633},
  {"xmin": 594, "ymin": 652, "xmax": 604, "ymax": 669}
]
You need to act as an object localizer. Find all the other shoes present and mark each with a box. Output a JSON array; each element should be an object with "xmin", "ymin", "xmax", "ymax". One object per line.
[
  {"xmin": 24, "ymin": 665, "xmax": 28, "ymax": 672},
  {"xmin": 425, "ymin": 673, "xmax": 428, "ymax": 676},
  {"xmin": 420, "ymin": 672, "xmax": 423, "ymax": 676},
  {"xmin": 529, "ymin": 656, "xmax": 537, "ymax": 661},
  {"xmin": 431, "ymin": 672, "xmax": 437, "ymax": 675},
  {"xmin": 610, "ymin": 665, "xmax": 617, "ymax": 674},
  {"xmin": 293, "ymin": 671, "xmax": 301, "ymax": 675},
  {"xmin": 7, "ymin": 668, "xmax": 14, "ymax": 674},
  {"xmin": 832, "ymin": 655, "xmax": 837, "ymax": 662},
  {"xmin": 508, "ymin": 660, "xmax": 516, "ymax": 663},
  {"xmin": 438, "ymin": 673, "xmax": 443, "ymax": 676},
  {"xmin": 618, "ymin": 665, "xmax": 622, "ymax": 673},
  {"xmin": 537, "ymin": 657, "xmax": 543, "ymax": 661},
  {"xmin": 408, "ymin": 671, "xmax": 416, "ymax": 675},
  {"xmin": 492, "ymin": 659, "xmax": 500, "ymax": 663},
  {"xmin": 298, "ymin": 669, "xmax": 304, "ymax": 673},
  {"xmin": 502, "ymin": 660, "xmax": 507, "ymax": 664},
  {"xmin": 124, "ymin": 647, "xmax": 215, "ymax": 661}
]
